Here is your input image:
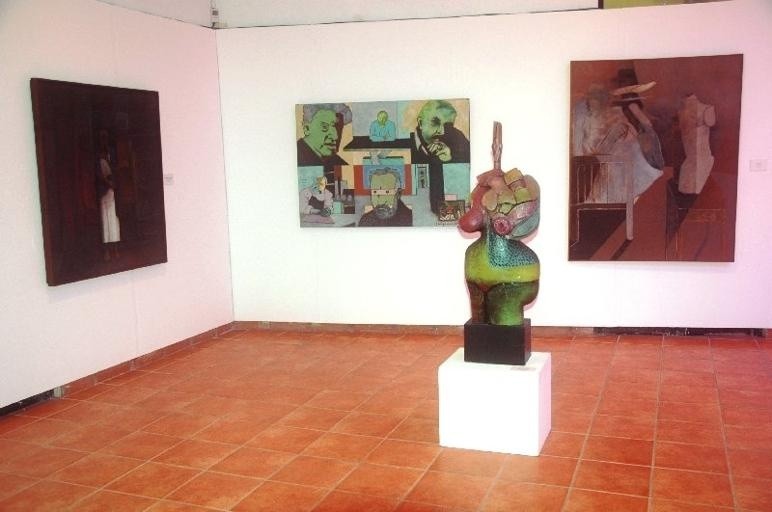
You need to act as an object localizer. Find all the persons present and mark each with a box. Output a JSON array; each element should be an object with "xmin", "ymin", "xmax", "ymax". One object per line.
[
  {"xmin": 356, "ymin": 167, "xmax": 412, "ymax": 227},
  {"xmin": 367, "ymin": 111, "xmax": 396, "ymax": 165},
  {"xmin": 298, "ymin": 104, "xmax": 352, "ymax": 166},
  {"xmin": 91, "ymin": 120, "xmax": 127, "ymax": 266},
  {"xmin": 674, "ymin": 88, "xmax": 717, "ymax": 195},
  {"xmin": 409, "ymin": 100, "xmax": 469, "ymax": 164},
  {"xmin": 302, "ymin": 172, "xmax": 337, "ymax": 227}
]
